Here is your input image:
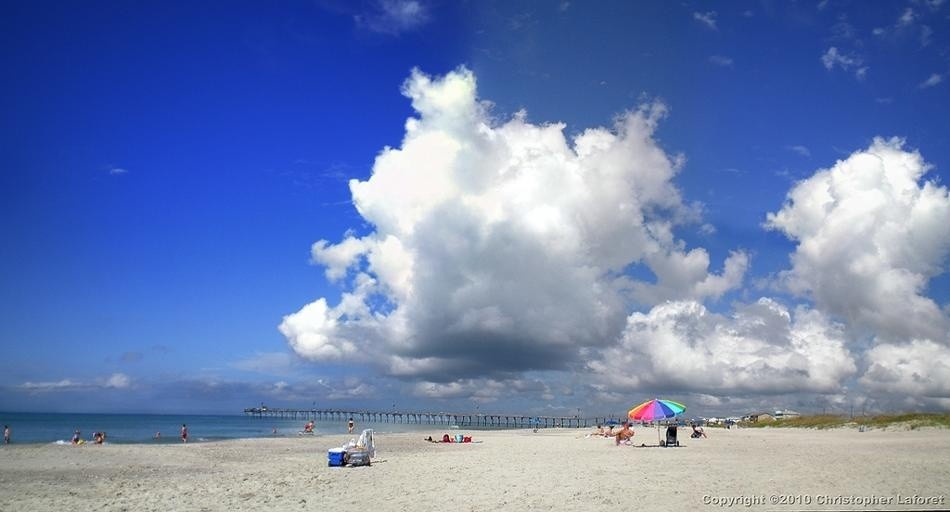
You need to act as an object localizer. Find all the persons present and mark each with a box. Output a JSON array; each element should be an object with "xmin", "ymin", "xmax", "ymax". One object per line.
[
  {"xmin": 304, "ymin": 421, "xmax": 315, "ymax": 433},
  {"xmin": 93, "ymin": 432, "xmax": 105, "ymax": 444},
  {"xmin": 3, "ymin": 425, "xmax": 10, "ymax": 444},
  {"xmin": 348, "ymin": 418, "xmax": 354, "ymax": 433},
  {"xmin": 181, "ymin": 424, "xmax": 188, "ymax": 444},
  {"xmin": 71, "ymin": 431, "xmax": 80, "ymax": 449},
  {"xmin": 591, "ymin": 424, "xmax": 635, "ymax": 446},
  {"xmin": 692, "ymin": 424, "xmax": 707, "ymax": 438}
]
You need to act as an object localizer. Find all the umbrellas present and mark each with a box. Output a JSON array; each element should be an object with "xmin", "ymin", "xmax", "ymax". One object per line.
[{"xmin": 627, "ymin": 398, "xmax": 686, "ymax": 443}]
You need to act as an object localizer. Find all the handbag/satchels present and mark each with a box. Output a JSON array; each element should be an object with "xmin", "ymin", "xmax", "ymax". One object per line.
[{"xmin": 443, "ymin": 434, "xmax": 472, "ymax": 443}]
